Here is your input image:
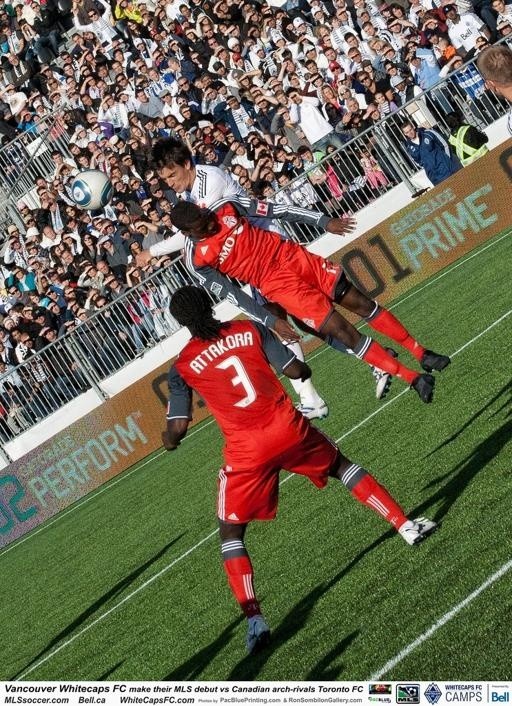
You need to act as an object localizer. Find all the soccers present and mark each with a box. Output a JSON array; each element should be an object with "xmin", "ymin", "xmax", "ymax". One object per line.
[{"xmin": 72, "ymin": 168, "xmax": 113, "ymax": 209}]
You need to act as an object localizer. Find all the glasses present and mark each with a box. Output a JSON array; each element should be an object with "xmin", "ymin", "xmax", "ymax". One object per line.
[
  {"xmin": 1, "ymin": 1, "xmax": 508, "ymax": 337},
  {"xmin": 1, "ymin": 48, "xmax": 369, "ymax": 345}
]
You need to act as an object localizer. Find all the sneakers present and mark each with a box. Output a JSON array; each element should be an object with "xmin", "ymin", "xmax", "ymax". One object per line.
[
  {"xmin": 399, "ymin": 521, "xmax": 438, "ymax": 545},
  {"xmin": 372, "ymin": 347, "xmax": 398, "ymax": 400},
  {"xmin": 246, "ymin": 615, "xmax": 270, "ymax": 654},
  {"xmin": 294, "ymin": 398, "xmax": 329, "ymax": 421},
  {"xmin": 411, "ymin": 372, "xmax": 435, "ymax": 403}
]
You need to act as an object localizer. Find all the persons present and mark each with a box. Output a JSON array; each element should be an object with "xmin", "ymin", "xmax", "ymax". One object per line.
[
  {"xmin": 0, "ymin": 0, "xmax": 512, "ymax": 444},
  {"xmin": 161, "ymin": 285, "xmax": 439, "ymax": 654}
]
